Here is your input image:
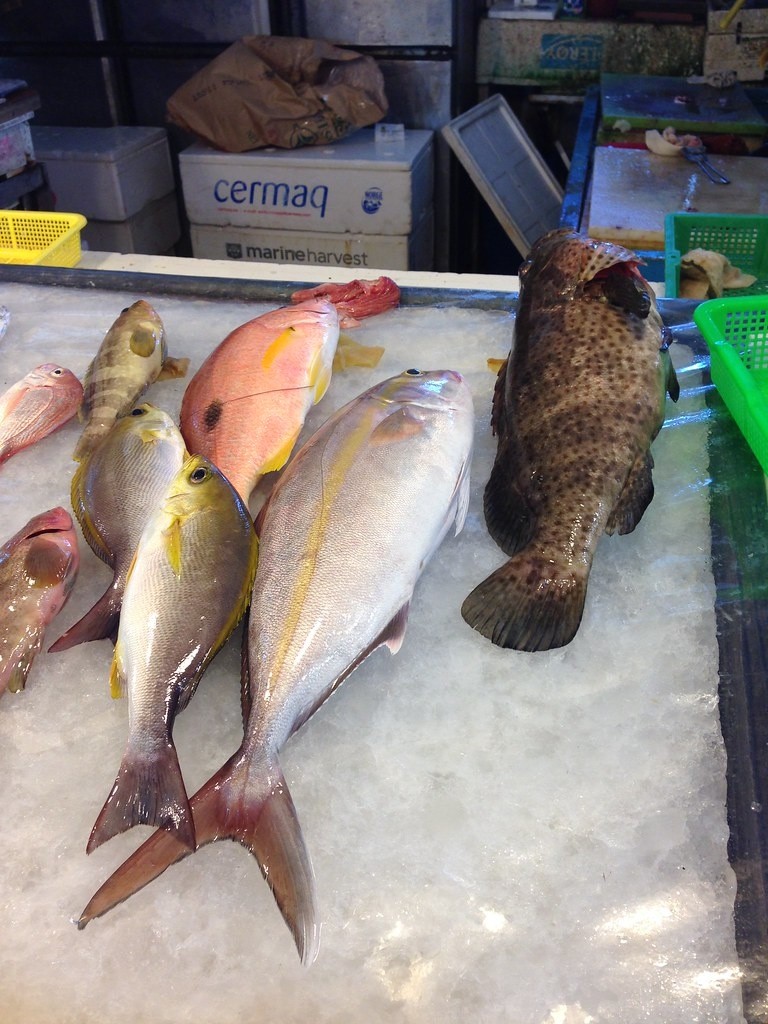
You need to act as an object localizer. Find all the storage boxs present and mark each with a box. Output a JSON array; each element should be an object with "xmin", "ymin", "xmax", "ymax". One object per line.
[
  {"xmin": 191, "ymin": 210, "xmax": 433, "ymax": 270},
  {"xmin": 179, "ymin": 130, "xmax": 435, "ymax": 232},
  {"xmin": 33, "ymin": 126, "xmax": 177, "ymax": 221},
  {"xmin": 81, "ymin": 190, "xmax": 181, "ymax": 254},
  {"xmin": 479, "ymin": 18, "xmax": 706, "ymax": 82}
]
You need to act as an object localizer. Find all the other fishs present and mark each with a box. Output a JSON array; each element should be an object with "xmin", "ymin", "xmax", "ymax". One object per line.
[{"xmin": 0, "ymin": 225, "xmax": 682, "ymax": 965}]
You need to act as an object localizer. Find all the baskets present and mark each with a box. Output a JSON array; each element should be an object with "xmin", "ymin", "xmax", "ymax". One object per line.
[
  {"xmin": 664, "ymin": 211, "xmax": 768, "ymax": 301},
  {"xmin": 0, "ymin": 209, "xmax": 89, "ymax": 269},
  {"xmin": 693, "ymin": 293, "xmax": 768, "ymax": 476}
]
